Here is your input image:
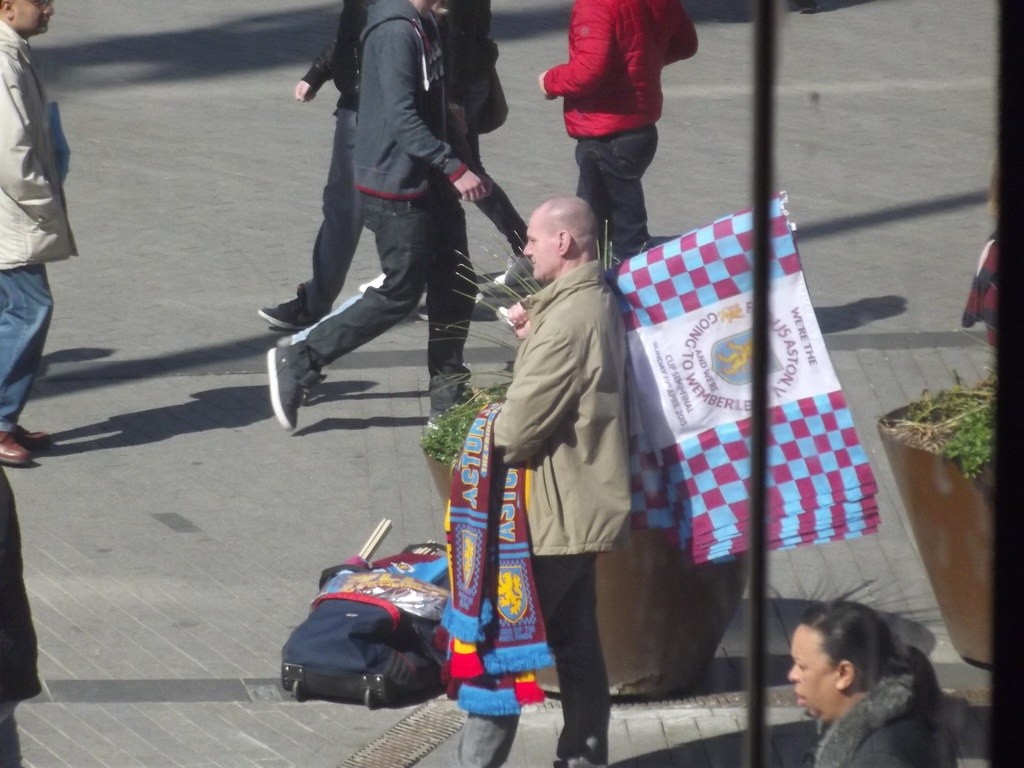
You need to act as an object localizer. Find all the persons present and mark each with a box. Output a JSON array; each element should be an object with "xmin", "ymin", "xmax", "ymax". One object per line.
[
  {"xmin": 0, "ymin": 466, "xmax": 41, "ymax": 768},
  {"xmin": 0, "ymin": 0, "xmax": 80, "ymax": 467},
  {"xmin": 787, "ymin": 600, "xmax": 960, "ymax": 768},
  {"xmin": 257, "ymin": 0, "xmax": 528, "ymax": 430},
  {"xmin": 457, "ymin": 197, "xmax": 632, "ymax": 768},
  {"xmin": 539, "ymin": 0, "xmax": 699, "ymax": 258}
]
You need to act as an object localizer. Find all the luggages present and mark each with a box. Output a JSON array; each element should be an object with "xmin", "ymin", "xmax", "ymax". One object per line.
[{"xmin": 274, "ymin": 539, "xmax": 449, "ymax": 712}]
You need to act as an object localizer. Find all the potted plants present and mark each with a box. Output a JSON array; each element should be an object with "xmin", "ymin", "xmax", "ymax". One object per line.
[
  {"xmin": 446, "ymin": 219, "xmax": 751, "ymax": 698},
  {"xmin": 878, "ymin": 361, "xmax": 994, "ymax": 665},
  {"xmin": 422, "ymin": 387, "xmax": 507, "ymax": 504}
]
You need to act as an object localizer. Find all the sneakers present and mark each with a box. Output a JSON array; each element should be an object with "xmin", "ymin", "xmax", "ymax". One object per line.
[
  {"xmin": 267, "ymin": 335, "xmax": 329, "ymax": 431},
  {"xmin": 359, "ymin": 274, "xmax": 386, "ymax": 292},
  {"xmin": 494, "ymin": 254, "xmax": 533, "ymax": 288},
  {"xmin": 256, "ymin": 297, "xmax": 319, "ymax": 331},
  {"xmin": 424, "ymin": 384, "xmax": 475, "ymax": 427},
  {"xmin": 417, "ymin": 304, "xmax": 431, "ymax": 322}
]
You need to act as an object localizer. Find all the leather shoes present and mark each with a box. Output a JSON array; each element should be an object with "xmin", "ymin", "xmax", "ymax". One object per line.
[
  {"xmin": 1, "ymin": 430, "xmax": 34, "ymax": 466},
  {"xmin": 11, "ymin": 426, "xmax": 52, "ymax": 451}
]
